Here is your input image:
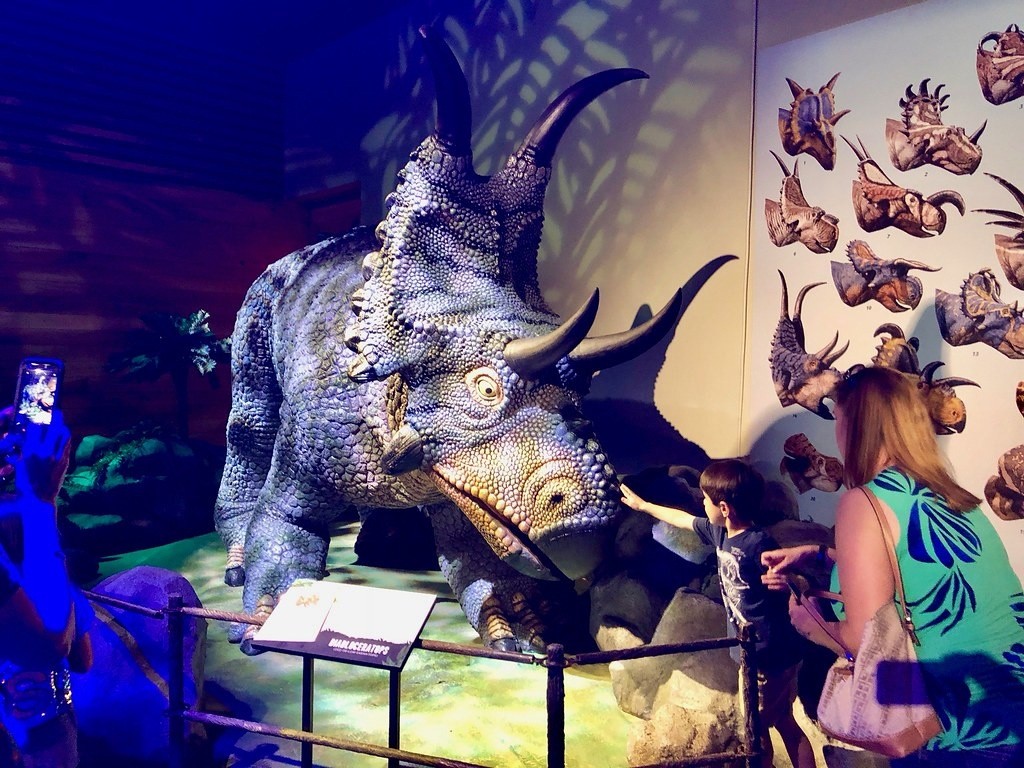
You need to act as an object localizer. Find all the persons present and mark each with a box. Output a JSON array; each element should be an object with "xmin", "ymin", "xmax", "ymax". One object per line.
[
  {"xmin": 620, "ymin": 459, "xmax": 818, "ymax": 768},
  {"xmin": 0, "ymin": 405, "xmax": 96, "ymax": 768},
  {"xmin": 760, "ymin": 365, "xmax": 1024, "ymax": 768}
]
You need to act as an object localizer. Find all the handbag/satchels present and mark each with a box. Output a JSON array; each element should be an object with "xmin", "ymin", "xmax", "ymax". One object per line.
[{"xmin": 810, "ymin": 484, "xmax": 938, "ymax": 755}]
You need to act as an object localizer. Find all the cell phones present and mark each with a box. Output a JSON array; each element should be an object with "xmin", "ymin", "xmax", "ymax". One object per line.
[
  {"xmin": 10, "ymin": 357, "xmax": 62, "ymax": 435},
  {"xmin": 784, "ymin": 576, "xmax": 804, "ymax": 605}
]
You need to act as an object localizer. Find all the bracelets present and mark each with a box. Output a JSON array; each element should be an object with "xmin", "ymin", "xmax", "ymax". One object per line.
[{"xmin": 818, "ymin": 544, "xmax": 828, "ymax": 568}]
